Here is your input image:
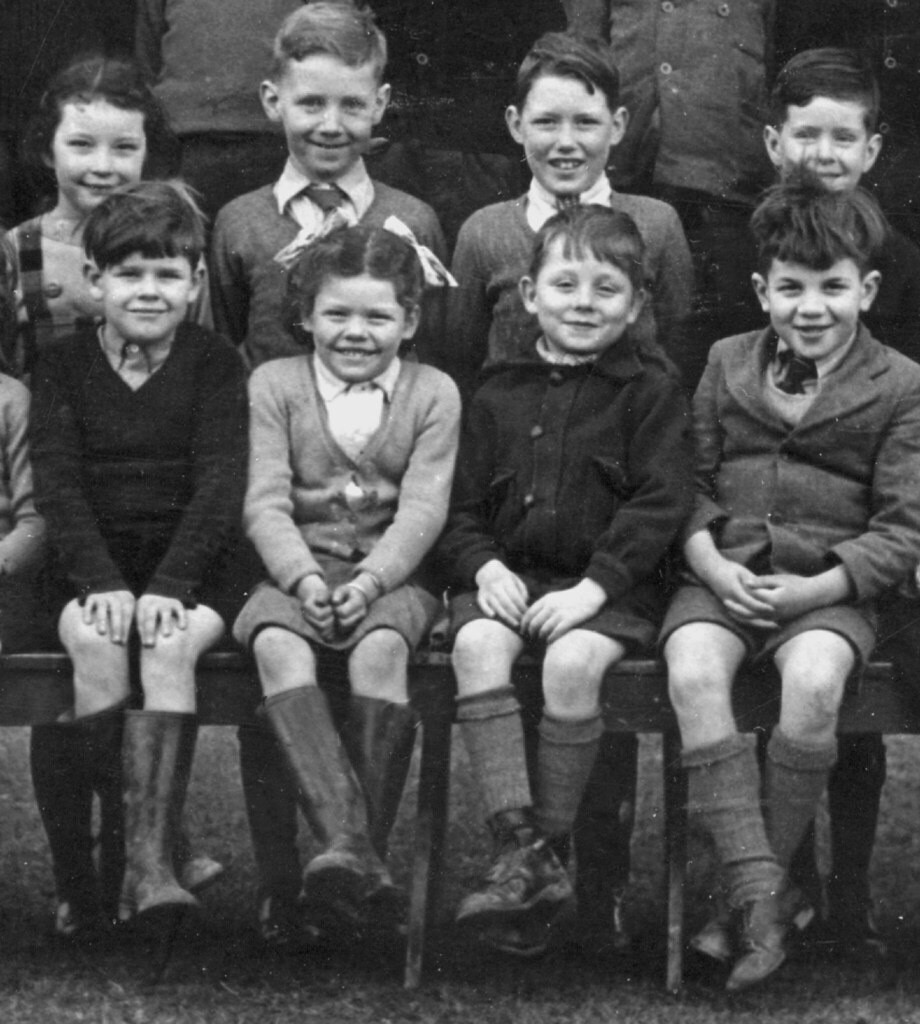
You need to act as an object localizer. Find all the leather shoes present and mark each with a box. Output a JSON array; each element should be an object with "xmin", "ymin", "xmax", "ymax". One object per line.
[{"xmin": 692, "ymin": 869, "xmax": 814, "ymax": 991}]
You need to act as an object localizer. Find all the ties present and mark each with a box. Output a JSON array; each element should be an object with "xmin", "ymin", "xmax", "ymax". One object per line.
[
  {"xmin": 556, "ymin": 194, "xmax": 580, "ymax": 212},
  {"xmin": 775, "ymin": 352, "xmax": 817, "ymax": 395},
  {"xmin": 303, "ymin": 186, "xmax": 345, "ymax": 222}
]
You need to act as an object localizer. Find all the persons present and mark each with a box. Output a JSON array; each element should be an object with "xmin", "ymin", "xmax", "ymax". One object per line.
[{"xmin": 0, "ymin": 0, "xmax": 919, "ymax": 973}]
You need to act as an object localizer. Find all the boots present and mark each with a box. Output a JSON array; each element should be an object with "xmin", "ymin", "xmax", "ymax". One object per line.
[
  {"xmin": 483, "ymin": 836, "xmax": 579, "ymax": 960},
  {"xmin": 263, "ymin": 694, "xmax": 412, "ymax": 923},
  {"xmin": 575, "ymin": 733, "xmax": 646, "ymax": 976},
  {"xmin": 293, "ymin": 697, "xmax": 415, "ymax": 954},
  {"xmin": 234, "ymin": 716, "xmax": 306, "ymax": 954},
  {"xmin": 32, "ymin": 696, "xmax": 223, "ymax": 927},
  {"xmin": 454, "ymin": 831, "xmax": 572, "ymax": 932},
  {"xmin": 755, "ymin": 734, "xmax": 886, "ymax": 981}
]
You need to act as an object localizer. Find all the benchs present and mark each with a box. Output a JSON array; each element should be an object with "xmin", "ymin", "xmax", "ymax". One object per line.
[{"xmin": 1, "ymin": 651, "xmax": 920, "ymax": 1024}]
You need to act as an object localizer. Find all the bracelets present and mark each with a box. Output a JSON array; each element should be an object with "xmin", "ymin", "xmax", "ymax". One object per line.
[{"xmin": 347, "ymin": 582, "xmax": 372, "ymax": 610}]
